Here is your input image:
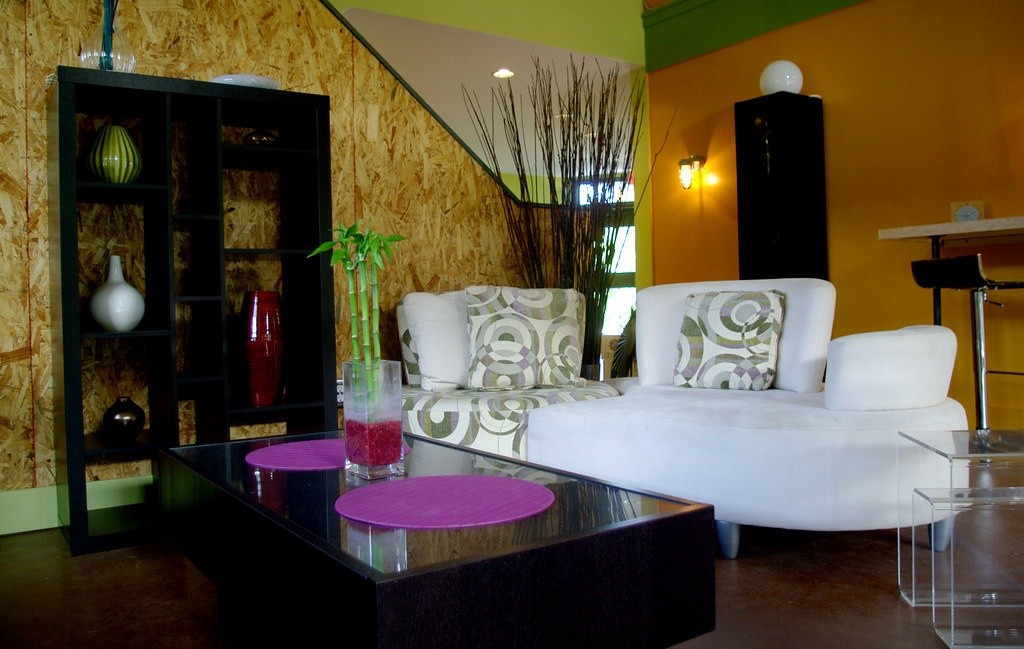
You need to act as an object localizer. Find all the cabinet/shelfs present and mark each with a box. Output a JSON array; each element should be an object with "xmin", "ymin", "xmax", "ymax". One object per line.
[
  {"xmin": 733, "ymin": 91, "xmax": 828, "ymax": 279},
  {"xmin": 44, "ymin": 63, "xmax": 339, "ymax": 555}
]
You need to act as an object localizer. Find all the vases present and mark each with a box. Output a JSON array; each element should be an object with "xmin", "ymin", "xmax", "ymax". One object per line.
[{"xmin": 306, "ymin": 217, "xmax": 406, "ymax": 478}]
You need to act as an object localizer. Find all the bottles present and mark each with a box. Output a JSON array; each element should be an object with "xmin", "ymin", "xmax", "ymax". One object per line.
[
  {"xmin": 91, "ymin": 255, "xmax": 145, "ymax": 332},
  {"xmin": 236, "ymin": 290, "xmax": 283, "ymax": 407},
  {"xmin": 85, "ymin": 125, "xmax": 143, "ymax": 185},
  {"xmin": 102, "ymin": 396, "xmax": 146, "ymax": 441}
]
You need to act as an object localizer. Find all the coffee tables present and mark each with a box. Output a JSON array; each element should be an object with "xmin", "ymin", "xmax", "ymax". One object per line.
[{"xmin": 153, "ymin": 428, "xmax": 715, "ymax": 648}]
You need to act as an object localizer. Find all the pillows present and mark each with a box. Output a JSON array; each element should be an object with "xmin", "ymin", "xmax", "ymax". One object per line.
[
  {"xmin": 405, "ymin": 290, "xmax": 467, "ymax": 391},
  {"xmin": 673, "ymin": 291, "xmax": 786, "ymax": 389},
  {"xmin": 466, "ymin": 285, "xmax": 587, "ymax": 388}
]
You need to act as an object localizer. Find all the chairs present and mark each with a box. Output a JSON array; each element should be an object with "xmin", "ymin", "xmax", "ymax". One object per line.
[
  {"xmin": 395, "ymin": 302, "xmax": 617, "ymax": 467},
  {"xmin": 526, "ymin": 278, "xmax": 972, "ymax": 561}
]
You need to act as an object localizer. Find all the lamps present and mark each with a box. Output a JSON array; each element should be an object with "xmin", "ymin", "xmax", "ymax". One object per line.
[{"xmin": 678, "ymin": 155, "xmax": 707, "ymax": 189}]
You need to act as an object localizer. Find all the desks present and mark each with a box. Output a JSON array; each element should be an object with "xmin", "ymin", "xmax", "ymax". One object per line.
[
  {"xmin": 894, "ymin": 429, "xmax": 1024, "ymax": 649},
  {"xmin": 878, "ymin": 215, "xmax": 1024, "ymax": 326}
]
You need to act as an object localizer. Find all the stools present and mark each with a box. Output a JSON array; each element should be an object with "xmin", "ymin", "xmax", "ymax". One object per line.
[{"xmin": 910, "ymin": 254, "xmax": 1024, "ymax": 506}]
